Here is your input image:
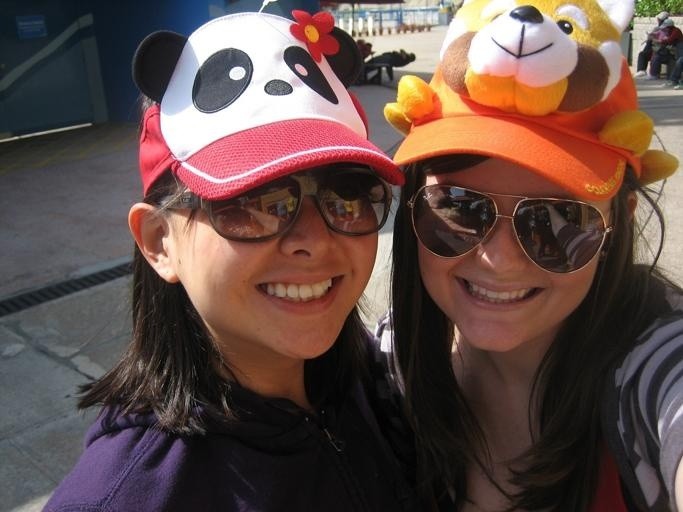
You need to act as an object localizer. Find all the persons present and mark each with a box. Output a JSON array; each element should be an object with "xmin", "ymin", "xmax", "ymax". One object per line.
[
  {"xmin": 320, "ymin": 191, "xmax": 364, "ymax": 232},
  {"xmin": 383, "ymin": 0, "xmax": 683, "ymax": 512},
  {"xmin": 41, "ymin": 9, "xmax": 457, "ymax": 512},
  {"xmin": 634, "ymin": 11, "xmax": 682, "ymax": 90},
  {"xmin": 240, "ymin": 178, "xmax": 299, "ymax": 236},
  {"xmin": 517, "ymin": 200, "xmax": 602, "ymax": 272}
]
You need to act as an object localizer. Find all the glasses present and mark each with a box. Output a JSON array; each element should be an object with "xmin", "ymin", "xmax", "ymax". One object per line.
[
  {"xmin": 155, "ymin": 171, "xmax": 394, "ymax": 243},
  {"xmin": 406, "ymin": 184, "xmax": 617, "ymax": 275}
]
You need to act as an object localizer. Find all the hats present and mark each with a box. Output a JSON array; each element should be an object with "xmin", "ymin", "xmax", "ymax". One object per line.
[
  {"xmin": 384, "ymin": 0, "xmax": 680, "ymax": 202},
  {"xmin": 132, "ymin": 8, "xmax": 406, "ymax": 203}
]
